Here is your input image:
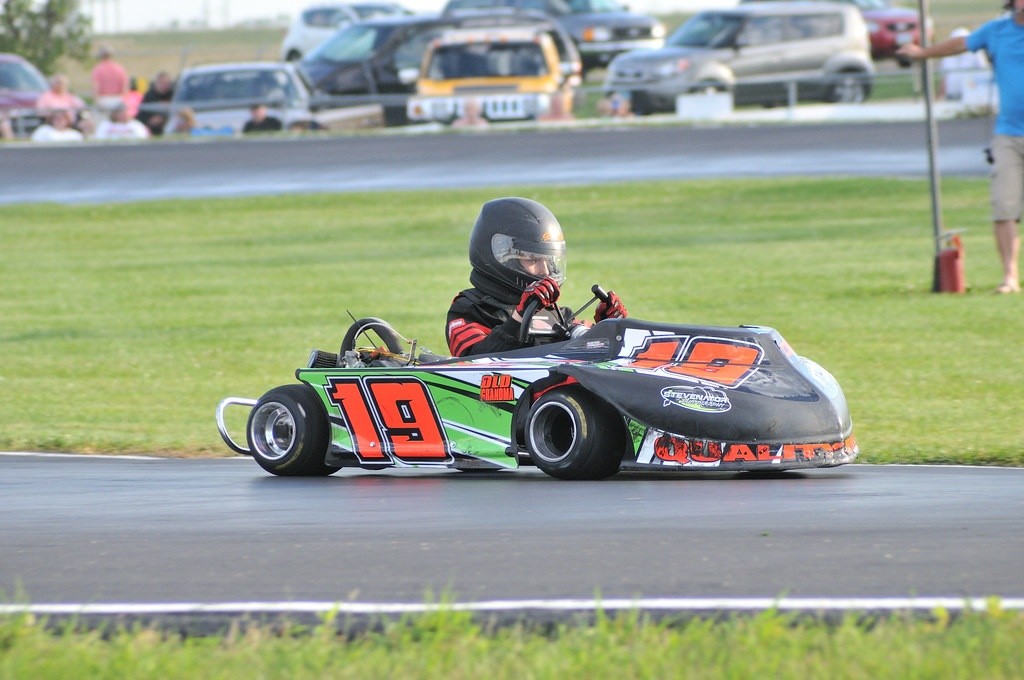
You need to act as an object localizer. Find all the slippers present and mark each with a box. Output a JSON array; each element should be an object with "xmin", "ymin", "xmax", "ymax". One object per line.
[{"xmin": 993, "ymin": 282, "xmax": 1021, "ymax": 296}]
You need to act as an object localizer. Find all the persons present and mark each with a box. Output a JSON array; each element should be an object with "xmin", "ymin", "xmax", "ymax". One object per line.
[
  {"xmin": 134, "ymin": 71, "xmax": 175, "ymax": 136},
  {"xmin": 174, "ymin": 107, "xmax": 197, "ymax": 137},
  {"xmin": 95, "ymin": 102, "xmax": 151, "ymax": 140},
  {"xmin": 451, "ymin": 96, "xmax": 489, "ymax": 130},
  {"xmin": 894, "ymin": 0, "xmax": 1024, "ymax": 294},
  {"xmin": 31, "ymin": 109, "xmax": 83, "ymax": 143},
  {"xmin": 89, "ymin": 45, "xmax": 129, "ymax": 98},
  {"xmin": 940, "ymin": 27, "xmax": 986, "ymax": 102},
  {"xmin": 536, "ymin": 93, "xmax": 575, "ymax": 121},
  {"xmin": 242, "ymin": 104, "xmax": 282, "ymax": 134},
  {"xmin": 38, "ymin": 74, "xmax": 81, "ymax": 127},
  {"xmin": 595, "ymin": 90, "xmax": 637, "ymax": 119},
  {"xmin": 445, "ymin": 197, "xmax": 627, "ymax": 361}
]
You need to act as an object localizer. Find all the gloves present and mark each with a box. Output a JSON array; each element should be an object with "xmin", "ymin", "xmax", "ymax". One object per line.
[
  {"xmin": 594, "ymin": 290, "xmax": 628, "ymax": 323},
  {"xmin": 515, "ymin": 276, "xmax": 561, "ymax": 318}
]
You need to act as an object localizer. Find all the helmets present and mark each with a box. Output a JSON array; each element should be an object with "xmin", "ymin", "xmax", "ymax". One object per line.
[{"xmin": 468, "ymin": 197, "xmax": 568, "ymax": 305}]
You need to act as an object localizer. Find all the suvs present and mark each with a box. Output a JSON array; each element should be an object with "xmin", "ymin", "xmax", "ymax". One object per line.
[
  {"xmin": 406, "ymin": 21, "xmax": 585, "ymax": 122},
  {"xmin": 273, "ymin": 0, "xmax": 667, "ymax": 128},
  {"xmin": 600, "ymin": 4, "xmax": 876, "ymax": 115},
  {"xmin": 737, "ymin": 0, "xmax": 934, "ymax": 69}
]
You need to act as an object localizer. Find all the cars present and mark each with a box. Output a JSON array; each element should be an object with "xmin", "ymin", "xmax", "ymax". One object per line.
[
  {"xmin": 164, "ymin": 60, "xmax": 315, "ymax": 137},
  {"xmin": 0, "ymin": 52, "xmax": 50, "ymax": 112}
]
